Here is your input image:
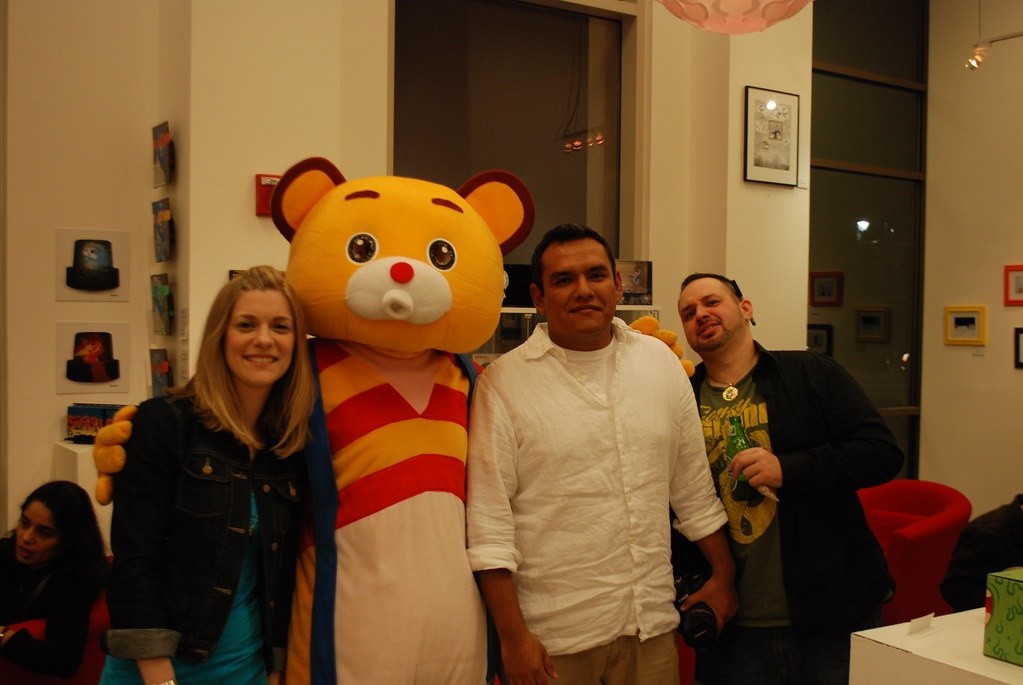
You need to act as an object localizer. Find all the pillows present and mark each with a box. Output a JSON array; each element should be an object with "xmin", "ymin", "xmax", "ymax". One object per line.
[{"xmin": 866, "ymin": 509, "xmax": 929, "ymax": 587}]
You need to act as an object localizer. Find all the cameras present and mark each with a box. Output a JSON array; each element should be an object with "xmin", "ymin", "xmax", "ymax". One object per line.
[{"xmin": 675, "ymin": 572, "xmax": 719, "ymax": 648}]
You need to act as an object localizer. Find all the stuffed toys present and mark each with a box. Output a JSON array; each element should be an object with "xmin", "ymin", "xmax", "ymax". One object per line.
[{"xmin": 92, "ymin": 155, "xmax": 696, "ymax": 685}]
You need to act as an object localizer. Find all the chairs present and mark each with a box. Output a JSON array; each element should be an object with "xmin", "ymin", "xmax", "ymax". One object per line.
[
  {"xmin": 0, "ymin": 555, "xmax": 114, "ymax": 685},
  {"xmin": 857, "ymin": 479, "xmax": 972, "ymax": 625}
]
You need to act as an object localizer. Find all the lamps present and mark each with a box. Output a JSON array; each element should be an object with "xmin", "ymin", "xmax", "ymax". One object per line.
[
  {"xmin": 555, "ymin": 42, "xmax": 606, "ymax": 154},
  {"xmin": 965, "ymin": 43, "xmax": 994, "ymax": 70}
]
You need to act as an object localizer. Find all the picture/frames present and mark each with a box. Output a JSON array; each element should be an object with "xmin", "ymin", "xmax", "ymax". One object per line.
[
  {"xmin": 808, "ymin": 324, "xmax": 833, "ymax": 357},
  {"xmin": 944, "ymin": 306, "xmax": 987, "ymax": 346},
  {"xmin": 854, "ymin": 306, "xmax": 889, "ymax": 343},
  {"xmin": 744, "ymin": 85, "xmax": 799, "ymax": 187},
  {"xmin": 1015, "ymin": 328, "xmax": 1023, "ymax": 369},
  {"xmin": 810, "ymin": 272, "xmax": 844, "ymax": 307},
  {"xmin": 1004, "ymin": 265, "xmax": 1023, "ymax": 307}
]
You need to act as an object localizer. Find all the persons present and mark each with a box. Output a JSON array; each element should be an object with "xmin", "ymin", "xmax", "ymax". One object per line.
[
  {"xmin": 465, "ymin": 224, "xmax": 737, "ymax": 685},
  {"xmin": 0, "ymin": 480, "xmax": 110, "ymax": 685},
  {"xmin": 666, "ymin": 274, "xmax": 905, "ymax": 685},
  {"xmin": 100, "ymin": 264, "xmax": 314, "ymax": 685}
]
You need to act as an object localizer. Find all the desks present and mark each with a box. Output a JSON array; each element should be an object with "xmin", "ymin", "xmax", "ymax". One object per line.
[
  {"xmin": 54, "ymin": 442, "xmax": 114, "ymax": 557},
  {"xmin": 848, "ymin": 608, "xmax": 1023, "ymax": 685}
]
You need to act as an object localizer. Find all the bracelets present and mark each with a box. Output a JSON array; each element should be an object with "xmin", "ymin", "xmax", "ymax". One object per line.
[{"xmin": 152, "ymin": 679, "xmax": 177, "ymax": 685}]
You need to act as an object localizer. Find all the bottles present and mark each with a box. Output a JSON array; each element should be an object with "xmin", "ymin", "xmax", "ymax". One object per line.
[{"xmin": 725, "ymin": 416, "xmax": 759, "ymax": 501}]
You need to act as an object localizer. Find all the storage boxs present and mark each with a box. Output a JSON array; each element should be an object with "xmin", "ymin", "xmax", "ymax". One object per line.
[{"xmin": 984, "ymin": 568, "xmax": 1023, "ymax": 667}]
